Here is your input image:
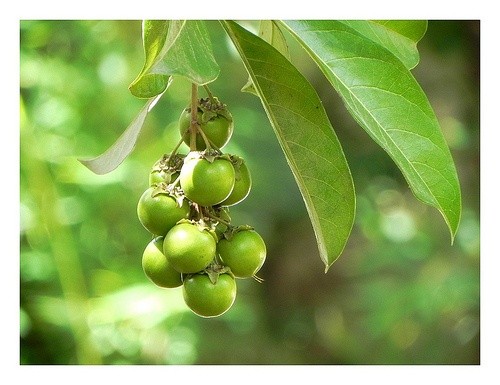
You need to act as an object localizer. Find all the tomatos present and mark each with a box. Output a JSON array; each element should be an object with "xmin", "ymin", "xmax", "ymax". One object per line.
[{"xmin": 137, "ymin": 97, "xmax": 267, "ymax": 318}]
